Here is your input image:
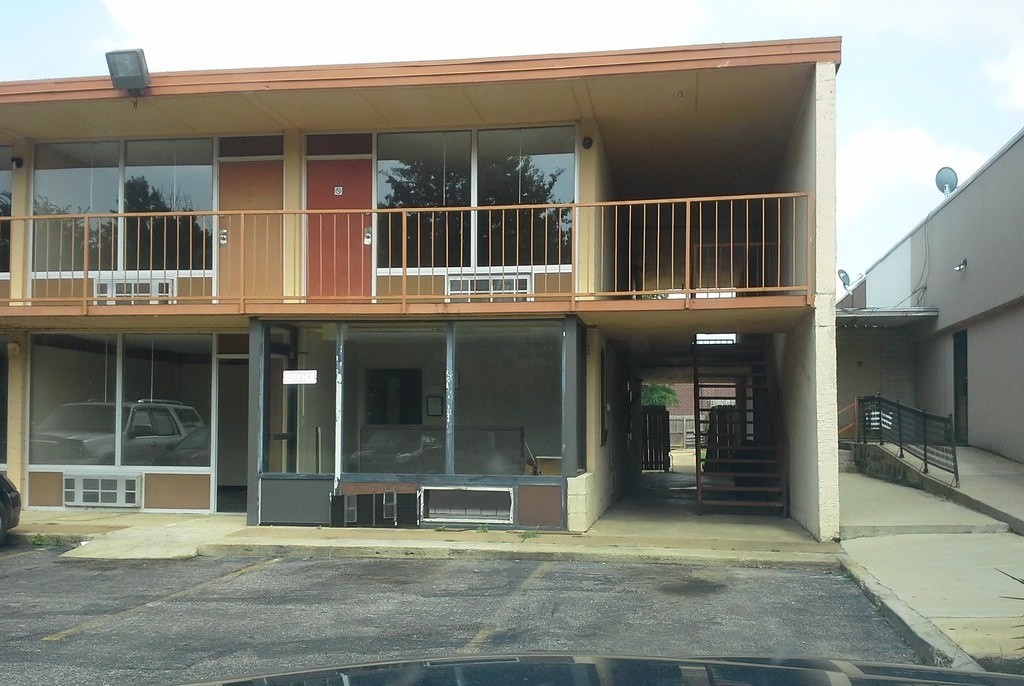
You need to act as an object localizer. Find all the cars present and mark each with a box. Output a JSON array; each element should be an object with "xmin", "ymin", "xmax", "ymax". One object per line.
[
  {"xmin": 348, "ymin": 427, "xmax": 449, "ymax": 474},
  {"xmin": 0, "ymin": 470, "xmax": 22, "ymax": 548}
]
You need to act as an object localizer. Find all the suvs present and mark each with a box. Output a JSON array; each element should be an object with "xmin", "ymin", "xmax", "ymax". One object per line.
[{"xmin": 30, "ymin": 398, "xmax": 212, "ymax": 466}]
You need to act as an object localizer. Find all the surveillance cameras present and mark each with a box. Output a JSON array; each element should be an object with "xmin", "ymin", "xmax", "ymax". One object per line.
[
  {"xmin": 954, "ymin": 266, "xmax": 964, "ymax": 271},
  {"xmin": 583, "ymin": 138, "xmax": 593, "ymax": 149}
]
